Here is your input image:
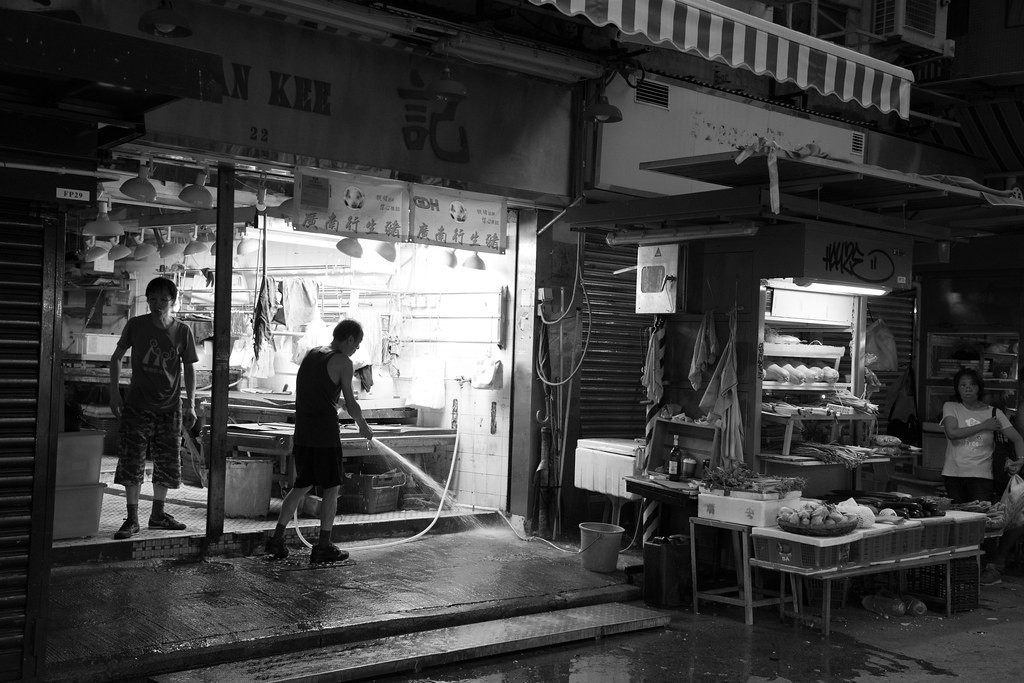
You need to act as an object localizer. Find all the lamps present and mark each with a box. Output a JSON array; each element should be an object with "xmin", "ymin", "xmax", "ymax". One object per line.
[
  {"xmin": 336, "ymin": 236, "xmax": 363, "ymax": 259},
  {"xmin": 582, "ymin": 76, "xmax": 623, "ymax": 125},
  {"xmin": 120, "ymin": 165, "xmax": 156, "ymax": 203},
  {"xmin": 237, "ymin": 230, "xmax": 263, "ymax": 255},
  {"xmin": 183, "ymin": 230, "xmax": 208, "ymax": 257},
  {"xmin": 138, "ymin": 0, "xmax": 193, "ymax": 39},
  {"xmin": 159, "ymin": 234, "xmax": 184, "ymax": 259},
  {"xmin": 278, "ymin": 198, "xmax": 294, "ymax": 220},
  {"xmin": 196, "ymin": 226, "xmax": 218, "ymax": 256},
  {"xmin": 430, "ymin": 57, "xmax": 468, "ymax": 102},
  {"xmin": 104, "ymin": 236, "xmax": 131, "ymax": 260},
  {"xmin": 606, "ymin": 223, "xmax": 758, "ymax": 246},
  {"xmin": 133, "ymin": 234, "xmax": 157, "ymax": 262},
  {"xmin": 442, "ymin": 247, "xmax": 456, "ymax": 269},
  {"xmin": 463, "ymin": 251, "xmax": 485, "ymax": 271},
  {"xmin": 375, "ymin": 241, "xmax": 399, "ymax": 262},
  {"xmin": 80, "ymin": 202, "xmax": 124, "ymax": 239},
  {"xmin": 84, "ymin": 235, "xmax": 106, "ymax": 263},
  {"xmin": 177, "ymin": 171, "xmax": 213, "ymax": 210}
]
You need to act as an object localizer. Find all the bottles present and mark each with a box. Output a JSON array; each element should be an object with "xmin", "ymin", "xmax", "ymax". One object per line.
[{"xmin": 668, "ymin": 435, "xmax": 683, "ymax": 482}]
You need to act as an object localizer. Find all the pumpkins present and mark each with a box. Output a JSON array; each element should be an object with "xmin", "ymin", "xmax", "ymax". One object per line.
[{"xmin": 763, "ymin": 357, "xmax": 839, "ymax": 385}]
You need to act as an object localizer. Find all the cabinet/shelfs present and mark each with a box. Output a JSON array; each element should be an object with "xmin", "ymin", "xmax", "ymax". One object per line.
[{"xmin": 745, "ymin": 277, "xmax": 866, "ymax": 497}]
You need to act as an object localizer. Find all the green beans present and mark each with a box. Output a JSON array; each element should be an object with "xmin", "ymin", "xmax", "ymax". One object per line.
[{"xmin": 765, "ymin": 440, "xmax": 868, "ymax": 469}]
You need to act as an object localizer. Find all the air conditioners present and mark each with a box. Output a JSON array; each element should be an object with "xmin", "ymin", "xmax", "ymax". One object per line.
[{"xmin": 870, "ymin": 0, "xmax": 950, "ymax": 58}]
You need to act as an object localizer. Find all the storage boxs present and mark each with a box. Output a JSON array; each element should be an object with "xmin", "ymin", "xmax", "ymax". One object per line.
[
  {"xmin": 751, "ymin": 523, "xmax": 864, "ymax": 573},
  {"xmin": 901, "ymin": 559, "xmax": 978, "ymax": 615},
  {"xmin": 908, "ymin": 516, "xmax": 955, "ymax": 551},
  {"xmin": 342, "ymin": 472, "xmax": 408, "ymax": 514},
  {"xmin": 75, "ymin": 333, "xmax": 132, "ymax": 358},
  {"xmin": 945, "ymin": 512, "xmax": 988, "ymax": 546},
  {"xmin": 410, "ymin": 405, "xmax": 443, "ymax": 429},
  {"xmin": 573, "ymin": 439, "xmax": 649, "ymax": 500},
  {"xmin": 850, "ymin": 521, "xmax": 925, "ymax": 563},
  {"xmin": 759, "ymin": 455, "xmax": 848, "ymax": 498},
  {"xmin": 697, "ymin": 490, "xmax": 804, "ymax": 528},
  {"xmin": 55, "ymin": 429, "xmax": 107, "ymax": 487},
  {"xmin": 53, "ymin": 486, "xmax": 107, "ymax": 539},
  {"xmin": 804, "ymin": 577, "xmax": 868, "ymax": 611}
]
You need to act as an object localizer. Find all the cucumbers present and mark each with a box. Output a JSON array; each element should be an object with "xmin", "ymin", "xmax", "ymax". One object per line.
[{"xmin": 821, "ymin": 489, "xmax": 945, "ymax": 519}]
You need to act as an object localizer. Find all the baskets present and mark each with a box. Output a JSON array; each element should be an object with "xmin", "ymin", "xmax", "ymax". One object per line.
[
  {"xmin": 745, "ymin": 507, "xmax": 987, "ymax": 573},
  {"xmin": 917, "ymin": 465, "xmax": 944, "ymax": 482}
]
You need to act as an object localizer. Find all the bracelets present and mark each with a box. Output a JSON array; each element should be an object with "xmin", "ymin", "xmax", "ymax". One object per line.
[{"xmin": 1016, "ymin": 456, "xmax": 1024, "ymax": 459}]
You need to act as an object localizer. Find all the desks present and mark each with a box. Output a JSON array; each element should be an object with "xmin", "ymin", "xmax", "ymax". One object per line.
[{"xmin": 749, "ymin": 550, "xmax": 984, "ymax": 639}]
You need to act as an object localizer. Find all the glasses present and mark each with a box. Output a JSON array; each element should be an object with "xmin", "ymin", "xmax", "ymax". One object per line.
[{"xmin": 146, "ymin": 296, "xmax": 175, "ymax": 307}]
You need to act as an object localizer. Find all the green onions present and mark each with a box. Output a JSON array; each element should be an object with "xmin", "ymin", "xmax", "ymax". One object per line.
[{"xmin": 759, "ymin": 394, "xmax": 832, "ymax": 417}]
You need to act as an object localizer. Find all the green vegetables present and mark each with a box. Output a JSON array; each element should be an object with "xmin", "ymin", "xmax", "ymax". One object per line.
[{"xmin": 704, "ymin": 459, "xmax": 807, "ymax": 497}]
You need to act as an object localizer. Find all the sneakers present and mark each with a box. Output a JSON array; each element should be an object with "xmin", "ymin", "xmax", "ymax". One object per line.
[
  {"xmin": 979, "ymin": 566, "xmax": 1003, "ymax": 586},
  {"xmin": 114, "ymin": 518, "xmax": 139, "ymax": 538},
  {"xmin": 265, "ymin": 536, "xmax": 290, "ymax": 556},
  {"xmin": 311, "ymin": 544, "xmax": 349, "ymax": 563},
  {"xmin": 148, "ymin": 512, "xmax": 187, "ymax": 529}
]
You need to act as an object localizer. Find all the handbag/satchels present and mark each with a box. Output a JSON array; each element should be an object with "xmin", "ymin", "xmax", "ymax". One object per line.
[
  {"xmin": 884, "ymin": 364, "xmax": 922, "ymax": 443},
  {"xmin": 1000, "ymin": 472, "xmax": 1024, "ymax": 528},
  {"xmin": 988, "ymin": 408, "xmax": 1016, "ymax": 496}
]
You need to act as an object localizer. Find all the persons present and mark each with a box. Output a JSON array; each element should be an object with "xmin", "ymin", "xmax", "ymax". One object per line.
[
  {"xmin": 108, "ymin": 277, "xmax": 200, "ymax": 538},
  {"xmin": 268, "ymin": 320, "xmax": 372, "ymax": 562},
  {"xmin": 937, "ymin": 368, "xmax": 1024, "ymax": 585}
]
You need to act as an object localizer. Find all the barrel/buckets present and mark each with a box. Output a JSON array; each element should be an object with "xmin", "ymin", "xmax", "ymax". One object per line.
[
  {"xmin": 224, "ymin": 457, "xmax": 274, "ymax": 519},
  {"xmin": 578, "ymin": 522, "xmax": 625, "ymax": 574}
]
